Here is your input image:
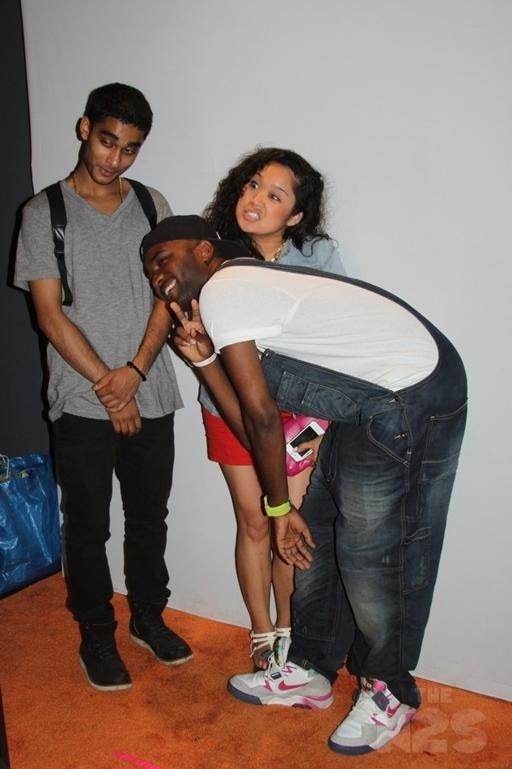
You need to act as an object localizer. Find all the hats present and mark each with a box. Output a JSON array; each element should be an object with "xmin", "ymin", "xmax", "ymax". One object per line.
[{"xmin": 139, "ymin": 215, "xmax": 241, "ymax": 259}]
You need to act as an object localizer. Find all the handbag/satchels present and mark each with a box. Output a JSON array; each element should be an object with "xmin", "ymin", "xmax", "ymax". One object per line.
[
  {"xmin": 282, "ymin": 412, "xmax": 332, "ymax": 477},
  {"xmin": 0, "ymin": 452, "xmax": 62, "ymax": 599}
]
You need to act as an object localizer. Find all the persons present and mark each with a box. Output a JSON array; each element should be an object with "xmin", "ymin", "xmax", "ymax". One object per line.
[
  {"xmin": 13, "ymin": 84, "xmax": 192, "ymax": 691},
  {"xmin": 199, "ymin": 149, "xmax": 347, "ymax": 670},
  {"xmin": 139, "ymin": 216, "xmax": 465, "ymax": 754}
]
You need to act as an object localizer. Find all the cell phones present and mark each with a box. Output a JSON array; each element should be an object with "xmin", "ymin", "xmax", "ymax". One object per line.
[{"xmin": 286, "ymin": 421, "xmax": 326, "ymax": 462}]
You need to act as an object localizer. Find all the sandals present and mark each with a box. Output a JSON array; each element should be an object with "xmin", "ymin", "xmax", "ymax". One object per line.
[{"xmin": 249, "ymin": 626, "xmax": 292, "ymax": 672}]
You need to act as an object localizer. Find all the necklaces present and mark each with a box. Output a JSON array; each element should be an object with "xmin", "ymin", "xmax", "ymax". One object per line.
[
  {"xmin": 71, "ymin": 174, "xmax": 123, "ymax": 205},
  {"xmin": 272, "ymin": 240, "xmax": 286, "ymax": 262}
]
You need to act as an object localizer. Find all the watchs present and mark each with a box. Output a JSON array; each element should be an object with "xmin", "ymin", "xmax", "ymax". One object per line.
[{"xmin": 261, "ymin": 495, "xmax": 296, "ymax": 519}]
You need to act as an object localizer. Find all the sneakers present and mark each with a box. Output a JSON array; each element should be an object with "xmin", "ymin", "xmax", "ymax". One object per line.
[
  {"xmin": 79, "ymin": 620, "xmax": 132, "ymax": 691},
  {"xmin": 227, "ymin": 661, "xmax": 334, "ymax": 711},
  {"xmin": 328, "ymin": 676, "xmax": 421, "ymax": 754},
  {"xmin": 129, "ymin": 604, "xmax": 193, "ymax": 665}
]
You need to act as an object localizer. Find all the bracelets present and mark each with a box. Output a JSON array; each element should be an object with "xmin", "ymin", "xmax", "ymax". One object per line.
[
  {"xmin": 126, "ymin": 360, "xmax": 147, "ymax": 382},
  {"xmin": 193, "ymin": 353, "xmax": 217, "ymax": 369}
]
